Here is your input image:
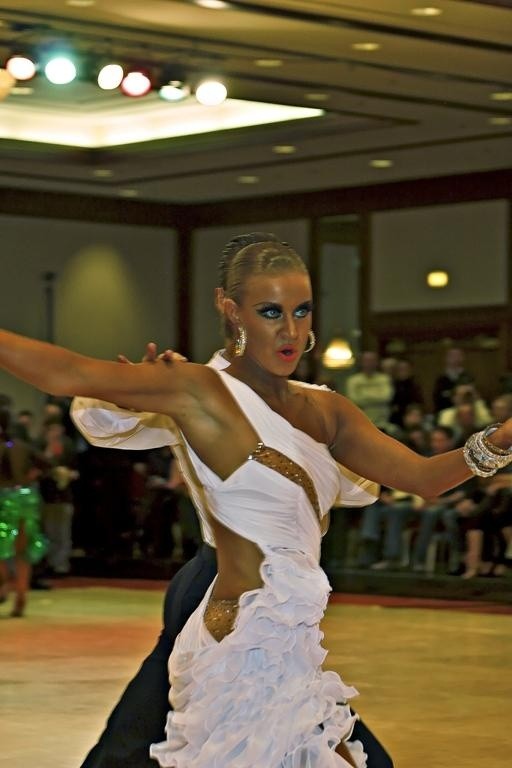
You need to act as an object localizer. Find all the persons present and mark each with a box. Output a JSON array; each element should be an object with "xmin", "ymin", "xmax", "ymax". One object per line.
[
  {"xmin": 1, "ymin": 242, "xmax": 511, "ymax": 766},
  {"xmin": 68, "ymin": 232, "xmax": 397, "ymax": 768}
]
